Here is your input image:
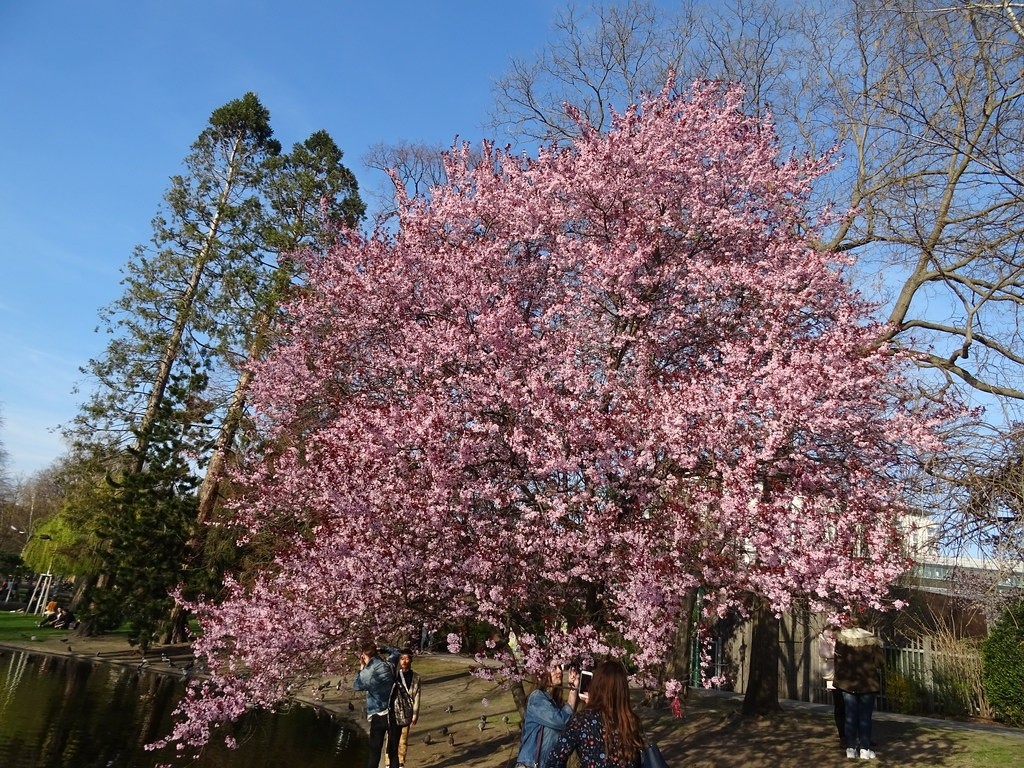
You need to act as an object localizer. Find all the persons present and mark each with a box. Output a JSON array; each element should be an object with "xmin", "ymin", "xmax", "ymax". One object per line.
[
  {"xmin": 1, "ymin": 575, "xmax": 77, "ymax": 631},
  {"xmin": 832, "ymin": 604, "xmax": 889, "ymax": 761},
  {"xmin": 352, "ymin": 640, "xmax": 402, "ymax": 768},
  {"xmin": 817, "ymin": 607, "xmax": 878, "ymax": 751},
  {"xmin": 384, "ymin": 647, "xmax": 422, "ymax": 767},
  {"xmin": 513, "ymin": 655, "xmax": 581, "ymax": 768},
  {"xmin": 546, "ymin": 659, "xmax": 648, "ymax": 767}
]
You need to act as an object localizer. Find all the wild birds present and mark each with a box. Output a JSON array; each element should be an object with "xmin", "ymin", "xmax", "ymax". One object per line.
[
  {"xmin": 312, "ymin": 676, "xmax": 348, "ymax": 701},
  {"xmin": 421, "ymin": 731, "xmax": 433, "ymax": 745},
  {"xmin": 39, "ymin": 534, "xmax": 52, "ymax": 541},
  {"xmin": 438, "ymin": 727, "xmax": 454, "ymax": 747},
  {"xmin": 502, "ymin": 715, "xmax": 509, "ymax": 725},
  {"xmin": 136, "ymin": 652, "xmax": 194, "ymax": 682},
  {"xmin": 444, "ymin": 704, "xmax": 453, "ymax": 714},
  {"xmin": 30, "ymin": 635, "xmax": 100, "ymax": 657},
  {"xmin": 478, "ymin": 715, "xmax": 486, "ymax": 733},
  {"xmin": 10, "ymin": 525, "xmax": 26, "ymax": 535},
  {"xmin": 349, "ymin": 702, "xmax": 355, "ymax": 713}
]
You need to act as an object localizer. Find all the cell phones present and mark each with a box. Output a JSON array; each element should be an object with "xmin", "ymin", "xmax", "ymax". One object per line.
[{"xmin": 578, "ymin": 669, "xmax": 594, "ymax": 699}]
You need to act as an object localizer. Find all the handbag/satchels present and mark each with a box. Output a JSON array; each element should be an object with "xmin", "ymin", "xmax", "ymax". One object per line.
[{"xmin": 639, "ymin": 744, "xmax": 669, "ymax": 768}]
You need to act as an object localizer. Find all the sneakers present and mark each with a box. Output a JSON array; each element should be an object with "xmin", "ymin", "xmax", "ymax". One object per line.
[
  {"xmin": 846, "ymin": 748, "xmax": 858, "ymax": 759},
  {"xmin": 860, "ymin": 748, "xmax": 875, "ymax": 760}
]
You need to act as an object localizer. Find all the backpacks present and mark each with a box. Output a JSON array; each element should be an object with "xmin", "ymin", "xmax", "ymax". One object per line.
[{"xmin": 386, "ymin": 662, "xmax": 412, "ymax": 732}]
